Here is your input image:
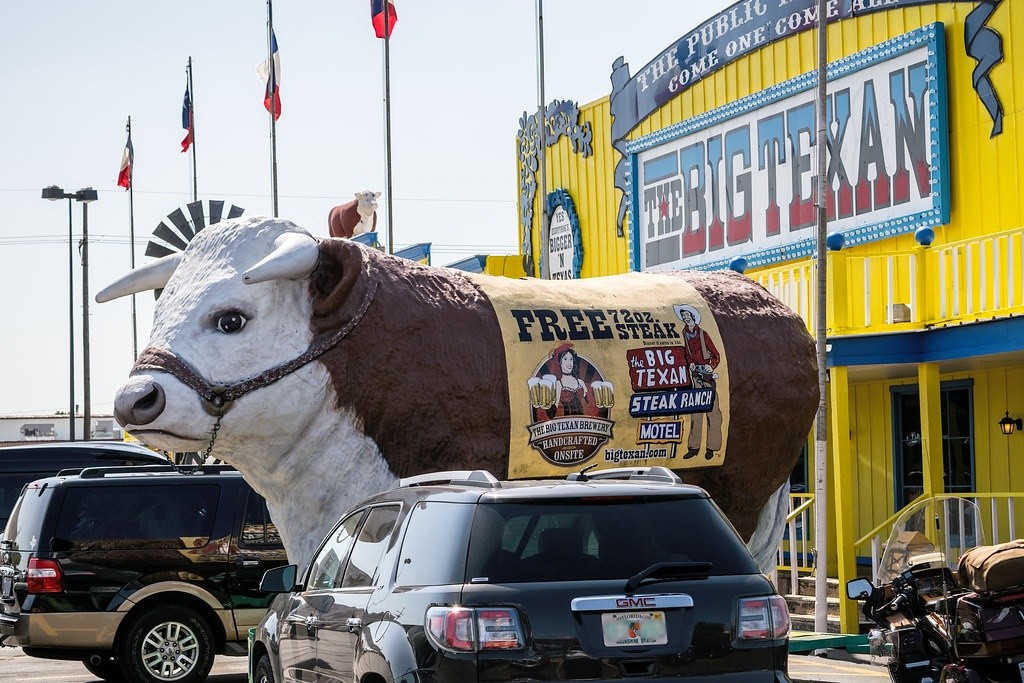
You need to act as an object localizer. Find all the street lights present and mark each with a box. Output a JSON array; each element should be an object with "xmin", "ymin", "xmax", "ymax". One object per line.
[{"xmin": 39, "ymin": 183, "xmax": 98, "ymax": 442}]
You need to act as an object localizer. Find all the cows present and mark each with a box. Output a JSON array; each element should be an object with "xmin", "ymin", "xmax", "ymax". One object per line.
[
  {"xmin": 327, "ymin": 191, "xmax": 382, "ymax": 239},
  {"xmin": 97, "ymin": 215, "xmax": 820, "ymax": 588}
]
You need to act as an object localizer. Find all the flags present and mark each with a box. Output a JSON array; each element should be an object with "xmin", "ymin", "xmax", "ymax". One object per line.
[
  {"xmin": 370, "ymin": 0, "xmax": 398, "ymax": 38},
  {"xmin": 263, "ymin": 27, "xmax": 282, "ymax": 121},
  {"xmin": 180, "ymin": 89, "xmax": 194, "ymax": 154},
  {"xmin": 117, "ymin": 135, "xmax": 134, "ymax": 192}
]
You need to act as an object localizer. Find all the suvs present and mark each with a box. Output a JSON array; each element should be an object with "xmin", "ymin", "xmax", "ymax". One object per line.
[
  {"xmin": 245, "ymin": 469, "xmax": 792, "ymax": 683},
  {"xmin": 0, "ymin": 444, "xmax": 177, "ymax": 545},
  {"xmin": 0, "ymin": 463, "xmax": 288, "ymax": 683}
]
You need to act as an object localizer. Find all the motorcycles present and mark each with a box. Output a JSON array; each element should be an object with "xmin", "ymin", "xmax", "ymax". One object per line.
[{"xmin": 843, "ymin": 494, "xmax": 1024, "ymax": 683}]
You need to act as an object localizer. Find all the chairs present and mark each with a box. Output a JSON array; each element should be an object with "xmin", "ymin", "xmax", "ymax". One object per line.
[{"xmin": 517, "ymin": 528, "xmax": 600, "ymax": 583}]
